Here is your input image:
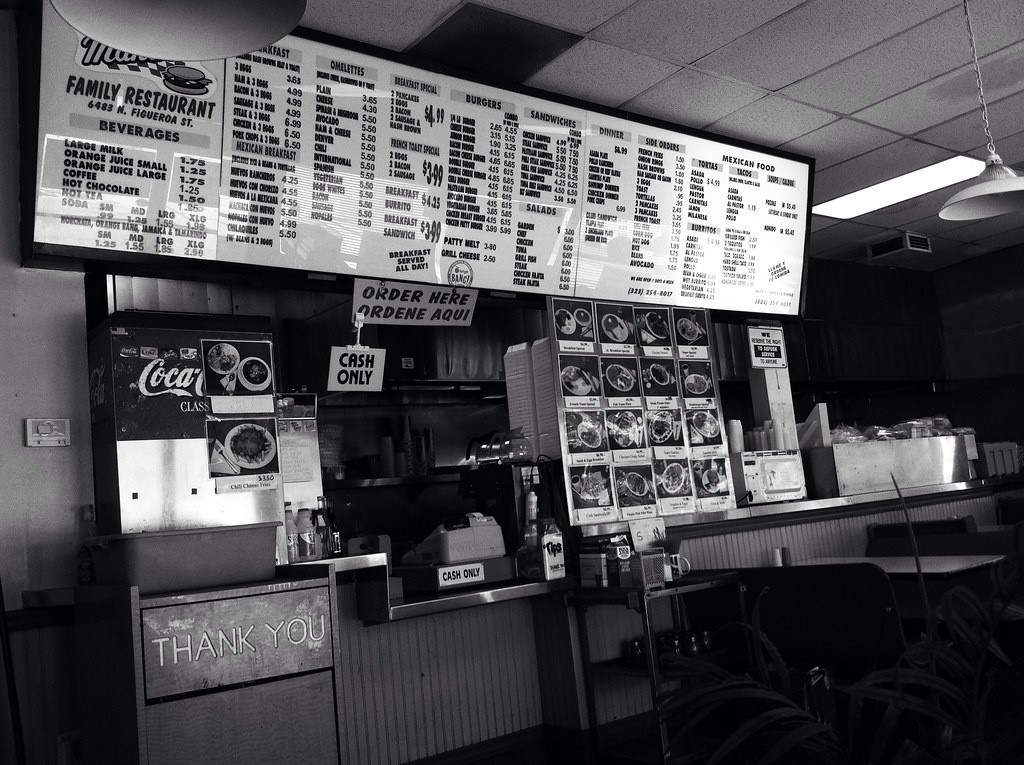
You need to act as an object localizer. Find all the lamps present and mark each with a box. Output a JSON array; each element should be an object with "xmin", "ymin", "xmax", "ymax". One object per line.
[{"xmin": 938, "ymin": 0, "xmax": 1024, "ymax": 221}]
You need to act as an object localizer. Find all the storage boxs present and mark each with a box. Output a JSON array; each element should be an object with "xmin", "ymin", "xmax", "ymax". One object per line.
[
  {"xmin": 975, "ymin": 441, "xmax": 1019, "ymax": 478},
  {"xmin": 807, "ymin": 435, "xmax": 978, "ymax": 497}
]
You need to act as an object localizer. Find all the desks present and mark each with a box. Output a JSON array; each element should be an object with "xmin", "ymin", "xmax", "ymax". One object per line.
[{"xmin": 787, "ymin": 553, "xmax": 1007, "ymax": 650}]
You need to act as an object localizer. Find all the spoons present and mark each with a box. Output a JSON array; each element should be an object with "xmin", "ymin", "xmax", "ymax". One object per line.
[{"xmin": 222, "ymin": 373, "xmax": 235, "ymax": 395}]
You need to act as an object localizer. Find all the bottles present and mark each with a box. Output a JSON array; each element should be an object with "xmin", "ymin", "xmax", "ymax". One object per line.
[
  {"xmin": 77, "ymin": 504, "xmax": 103, "ymax": 582},
  {"xmin": 630, "ymin": 631, "xmax": 713, "ymax": 657},
  {"xmin": 296, "ymin": 508, "xmax": 316, "ymax": 562},
  {"xmin": 524, "ymin": 518, "xmax": 566, "ymax": 582},
  {"xmin": 311, "ymin": 495, "xmax": 330, "ymax": 560},
  {"xmin": 325, "ymin": 497, "xmax": 342, "ymax": 558},
  {"xmin": 285, "ymin": 501, "xmax": 300, "ymax": 564}
]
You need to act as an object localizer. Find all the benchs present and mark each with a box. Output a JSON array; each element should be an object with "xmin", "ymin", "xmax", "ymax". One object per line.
[{"xmin": 664, "ymin": 498, "xmax": 1024, "ymax": 670}]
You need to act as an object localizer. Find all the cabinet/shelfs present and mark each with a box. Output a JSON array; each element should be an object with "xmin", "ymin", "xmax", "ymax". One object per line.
[
  {"xmin": 570, "ymin": 571, "xmax": 752, "ymax": 765},
  {"xmin": 64, "ymin": 588, "xmax": 351, "ymax": 765},
  {"xmin": 301, "ymin": 377, "xmax": 515, "ymax": 550}
]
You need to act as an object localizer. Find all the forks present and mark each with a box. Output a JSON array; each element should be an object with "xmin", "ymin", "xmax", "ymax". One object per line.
[{"xmin": 212, "ymin": 441, "xmax": 239, "ymax": 475}]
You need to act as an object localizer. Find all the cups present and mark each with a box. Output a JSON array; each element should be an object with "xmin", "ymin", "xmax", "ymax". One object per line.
[
  {"xmin": 689, "ymin": 310, "xmax": 696, "ymax": 322},
  {"xmin": 574, "ymin": 308, "xmax": 592, "ymax": 326},
  {"xmin": 283, "ymin": 397, "xmax": 294, "ymax": 412},
  {"xmin": 694, "ymin": 464, "xmax": 703, "ymax": 480},
  {"xmin": 682, "ymin": 364, "xmax": 690, "ymax": 379},
  {"xmin": 772, "ymin": 547, "xmax": 792, "ymax": 566},
  {"xmin": 334, "ymin": 465, "xmax": 345, "ymax": 480},
  {"xmin": 670, "ymin": 553, "xmax": 691, "ymax": 580},
  {"xmin": 571, "ymin": 475, "xmax": 582, "ymax": 494}
]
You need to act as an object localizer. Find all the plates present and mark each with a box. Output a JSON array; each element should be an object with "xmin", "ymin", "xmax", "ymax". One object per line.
[
  {"xmin": 224, "ymin": 423, "xmax": 276, "ymax": 469},
  {"xmin": 662, "ymin": 462, "xmax": 685, "ymax": 494},
  {"xmin": 241, "ymin": 358, "xmax": 269, "ymax": 386},
  {"xmin": 677, "ymin": 317, "xmax": 699, "ymax": 340},
  {"xmin": 701, "ymin": 470, "xmax": 721, "ymax": 494},
  {"xmin": 577, "ymin": 422, "xmax": 602, "ymax": 448},
  {"xmin": 692, "ymin": 411, "xmax": 720, "ymax": 438},
  {"xmin": 645, "ymin": 312, "xmax": 669, "ymax": 340},
  {"xmin": 615, "ymin": 411, "xmax": 637, "ymax": 447},
  {"xmin": 685, "ymin": 373, "xmax": 708, "ymax": 394},
  {"xmin": 207, "ymin": 343, "xmax": 240, "ymax": 374},
  {"xmin": 555, "ymin": 309, "xmax": 576, "ymax": 334},
  {"xmin": 606, "ymin": 364, "xmax": 634, "ymax": 392},
  {"xmin": 648, "ymin": 411, "xmax": 674, "ymax": 444},
  {"xmin": 237, "ymin": 357, "xmax": 271, "ymax": 392},
  {"xmin": 562, "ymin": 366, "xmax": 592, "ymax": 395},
  {"xmin": 650, "ymin": 363, "xmax": 669, "ymax": 385},
  {"xmin": 626, "ymin": 472, "xmax": 649, "ymax": 496},
  {"xmin": 602, "ymin": 314, "xmax": 628, "ymax": 343},
  {"xmin": 209, "ymin": 438, "xmax": 241, "ymax": 475}
]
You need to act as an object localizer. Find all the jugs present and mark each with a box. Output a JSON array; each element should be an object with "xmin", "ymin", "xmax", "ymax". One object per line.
[
  {"xmin": 487, "ymin": 426, "xmax": 535, "ymax": 463},
  {"xmin": 466, "ymin": 431, "xmax": 503, "ymax": 467}
]
